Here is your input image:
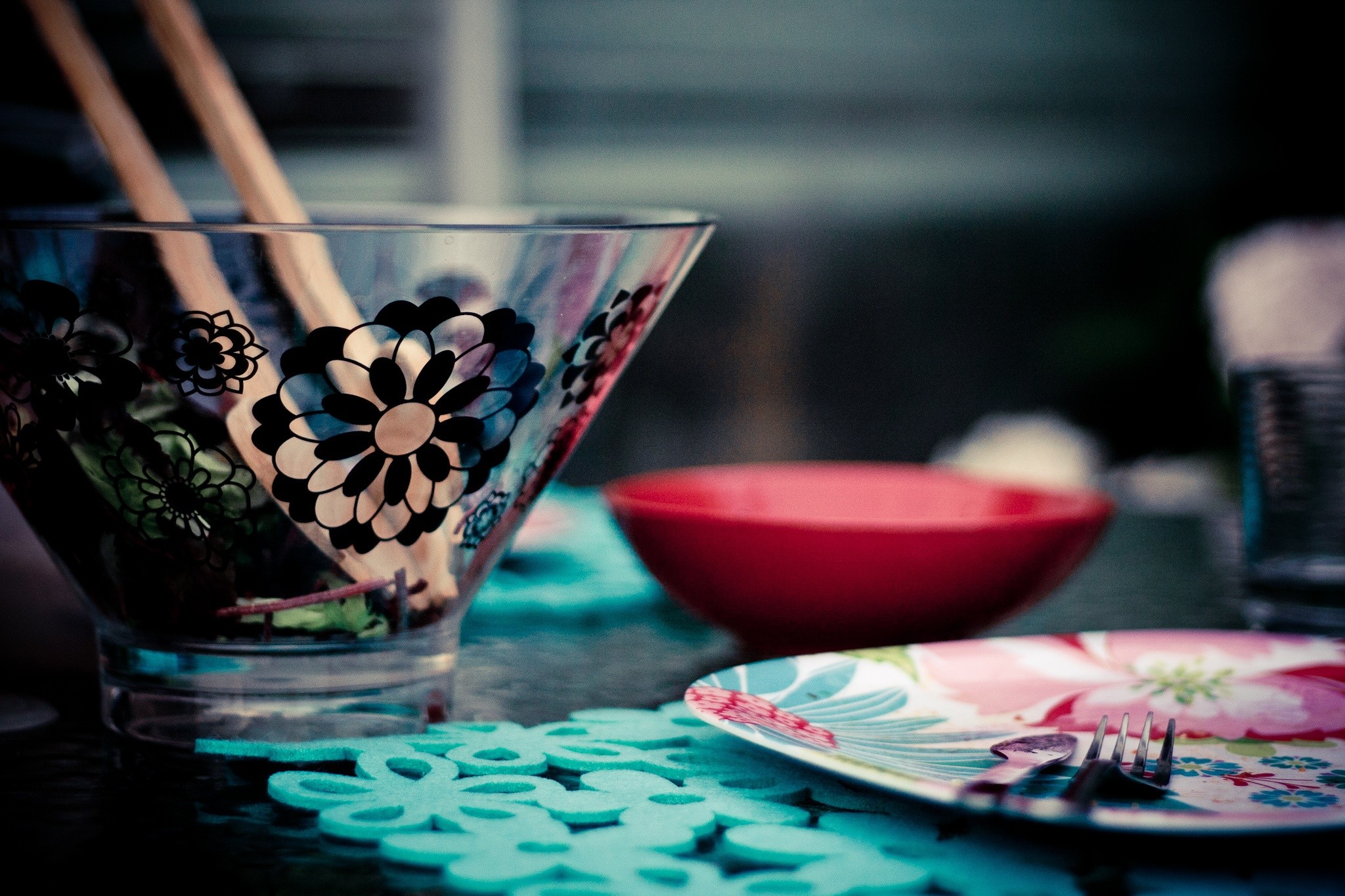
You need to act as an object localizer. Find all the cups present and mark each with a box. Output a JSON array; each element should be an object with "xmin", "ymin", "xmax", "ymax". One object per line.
[{"xmin": 1239, "ymin": 359, "xmax": 1345, "ymax": 625}]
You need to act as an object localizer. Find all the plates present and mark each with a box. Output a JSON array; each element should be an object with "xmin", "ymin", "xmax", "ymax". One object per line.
[{"xmin": 680, "ymin": 628, "xmax": 1344, "ymax": 831}]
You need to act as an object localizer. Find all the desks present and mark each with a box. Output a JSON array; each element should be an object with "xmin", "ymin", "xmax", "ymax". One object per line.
[{"xmin": 0, "ymin": 517, "xmax": 1345, "ymax": 896}]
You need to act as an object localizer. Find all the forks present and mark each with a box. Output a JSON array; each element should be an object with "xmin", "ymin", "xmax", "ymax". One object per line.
[{"xmin": 1059, "ymin": 711, "xmax": 1176, "ymax": 805}]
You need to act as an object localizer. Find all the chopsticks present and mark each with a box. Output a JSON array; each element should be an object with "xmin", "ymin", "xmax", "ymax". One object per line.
[{"xmin": 31, "ymin": 2, "xmax": 459, "ymax": 606}]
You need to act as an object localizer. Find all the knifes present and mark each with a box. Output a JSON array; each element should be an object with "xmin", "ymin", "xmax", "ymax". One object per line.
[{"xmin": 968, "ymin": 734, "xmax": 1076, "ymax": 787}]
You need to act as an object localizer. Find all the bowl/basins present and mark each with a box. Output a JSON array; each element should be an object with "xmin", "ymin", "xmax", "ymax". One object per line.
[
  {"xmin": 604, "ymin": 460, "xmax": 1119, "ymax": 655},
  {"xmin": 0, "ymin": 204, "xmax": 728, "ymax": 744}
]
